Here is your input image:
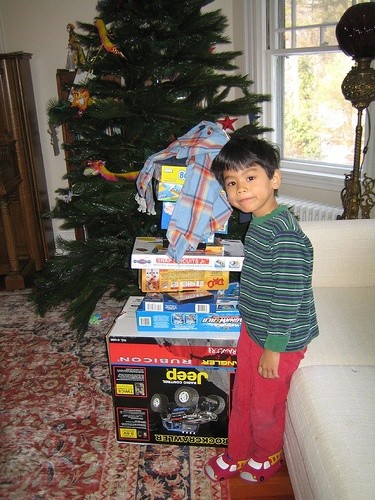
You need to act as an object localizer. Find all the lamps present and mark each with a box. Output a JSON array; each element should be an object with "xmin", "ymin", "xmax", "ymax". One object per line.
[{"xmin": 334, "ymin": 1, "xmax": 375, "ymax": 219}]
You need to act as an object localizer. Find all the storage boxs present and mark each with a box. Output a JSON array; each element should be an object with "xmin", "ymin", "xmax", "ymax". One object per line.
[
  {"xmin": 153, "ymin": 179, "xmax": 227, "ymax": 201},
  {"xmin": 104, "ymin": 295, "xmax": 242, "ymax": 448},
  {"xmin": 131, "ymin": 236, "xmax": 245, "ymax": 271},
  {"xmin": 153, "ymin": 160, "xmax": 187, "ymax": 183},
  {"xmin": 136, "ymin": 281, "xmax": 242, "ymax": 332},
  {"xmin": 160, "ymin": 202, "xmax": 229, "ymax": 235},
  {"xmin": 138, "ymin": 269, "xmax": 230, "ymax": 293},
  {"xmin": 142, "ymin": 291, "xmax": 217, "ymax": 312}
]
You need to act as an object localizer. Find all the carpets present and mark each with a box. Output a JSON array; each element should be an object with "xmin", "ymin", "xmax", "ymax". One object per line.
[{"xmin": 0, "ymin": 285, "xmax": 230, "ymax": 500}]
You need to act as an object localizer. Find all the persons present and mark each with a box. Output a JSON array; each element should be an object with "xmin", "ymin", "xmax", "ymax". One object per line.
[{"xmin": 203, "ymin": 135, "xmax": 321, "ymax": 484}]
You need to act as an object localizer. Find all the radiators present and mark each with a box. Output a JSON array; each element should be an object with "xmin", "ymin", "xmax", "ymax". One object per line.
[{"xmin": 276, "ymin": 193, "xmax": 344, "ymax": 220}]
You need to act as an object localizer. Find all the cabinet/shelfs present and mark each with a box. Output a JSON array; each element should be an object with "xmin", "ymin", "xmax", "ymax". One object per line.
[{"xmin": 0, "ymin": 50, "xmax": 48, "ymax": 288}]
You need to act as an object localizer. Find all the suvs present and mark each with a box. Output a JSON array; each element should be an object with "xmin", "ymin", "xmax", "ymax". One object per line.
[{"xmin": 150, "ymin": 386, "xmax": 226, "ymax": 435}]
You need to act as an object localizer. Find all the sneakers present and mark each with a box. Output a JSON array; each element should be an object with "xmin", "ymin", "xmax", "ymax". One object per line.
[
  {"xmin": 204, "ymin": 449, "xmax": 253, "ymax": 482},
  {"xmin": 239, "ymin": 446, "xmax": 283, "ymax": 483}
]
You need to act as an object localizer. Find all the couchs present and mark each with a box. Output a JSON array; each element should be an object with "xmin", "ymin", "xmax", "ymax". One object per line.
[{"xmin": 282, "ymin": 218, "xmax": 374, "ymax": 500}]
